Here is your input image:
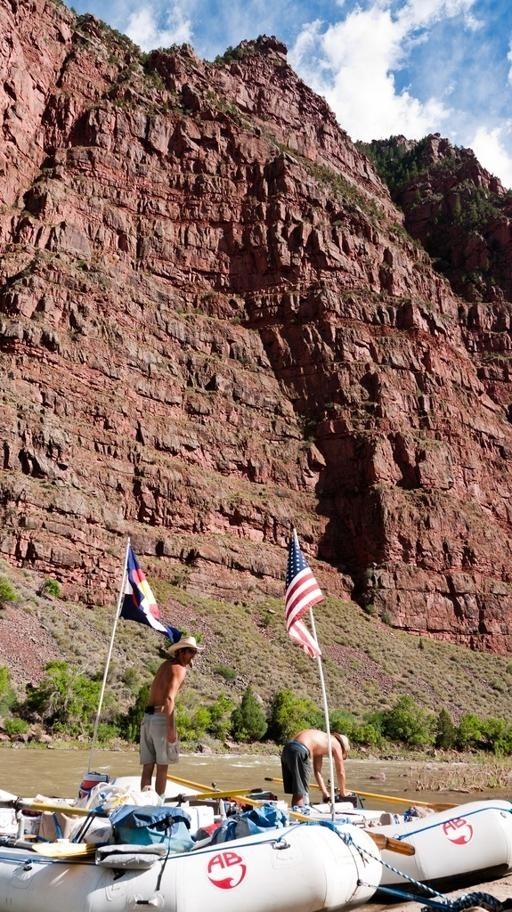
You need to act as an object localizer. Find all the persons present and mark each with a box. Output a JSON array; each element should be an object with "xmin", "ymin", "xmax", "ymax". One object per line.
[
  {"xmin": 138, "ymin": 636, "xmax": 205, "ymax": 797},
  {"xmin": 280, "ymin": 728, "xmax": 351, "ymax": 809}
]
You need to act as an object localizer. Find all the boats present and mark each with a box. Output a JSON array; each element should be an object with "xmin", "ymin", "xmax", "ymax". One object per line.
[
  {"xmin": 0, "ymin": 788, "xmax": 381, "ymax": 912},
  {"xmin": 77, "ymin": 776, "xmax": 511, "ymax": 904}
]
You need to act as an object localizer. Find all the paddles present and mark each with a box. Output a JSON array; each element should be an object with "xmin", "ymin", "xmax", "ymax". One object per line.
[
  {"xmin": 265, "ymin": 777, "xmax": 460, "ymax": 812},
  {"xmin": 32, "ymin": 840, "xmax": 109, "ymax": 858}
]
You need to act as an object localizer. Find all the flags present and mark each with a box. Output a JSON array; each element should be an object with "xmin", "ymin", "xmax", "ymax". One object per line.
[
  {"xmin": 283, "ymin": 532, "xmax": 326, "ymax": 660},
  {"xmin": 116, "ymin": 541, "xmax": 184, "ymax": 644}
]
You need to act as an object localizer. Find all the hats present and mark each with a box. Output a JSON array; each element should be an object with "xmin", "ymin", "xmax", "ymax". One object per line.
[
  {"xmin": 331, "ymin": 732, "xmax": 350, "ymax": 760},
  {"xmin": 168, "ymin": 636, "xmax": 205, "ymax": 656}
]
